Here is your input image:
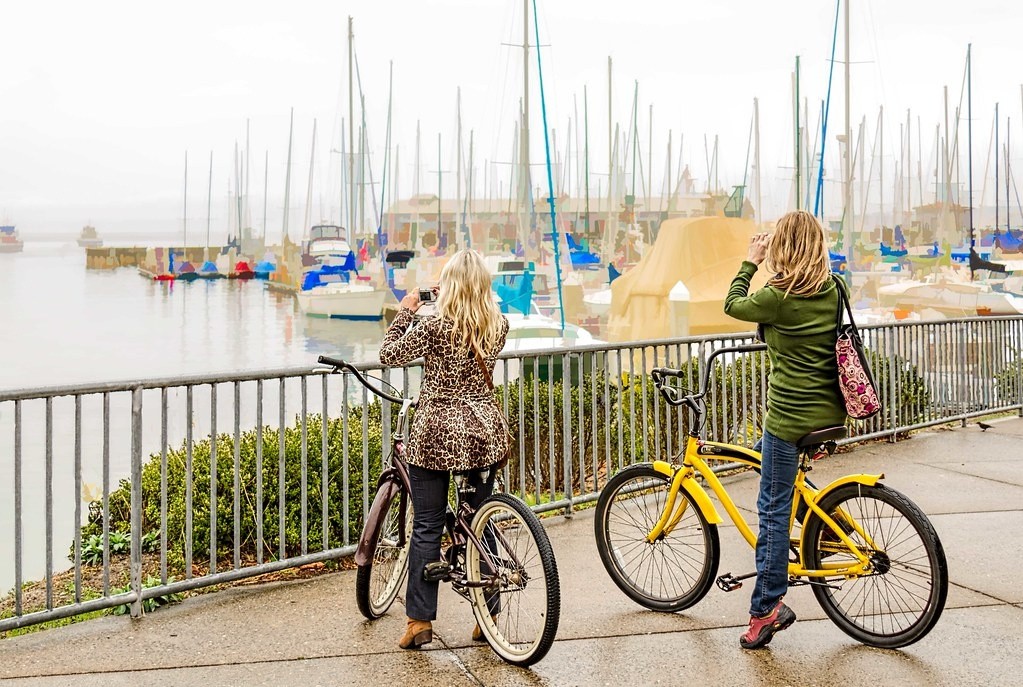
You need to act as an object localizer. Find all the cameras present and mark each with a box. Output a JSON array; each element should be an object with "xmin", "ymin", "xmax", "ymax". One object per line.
[{"xmin": 419, "ymin": 290, "xmax": 437, "ymax": 305}]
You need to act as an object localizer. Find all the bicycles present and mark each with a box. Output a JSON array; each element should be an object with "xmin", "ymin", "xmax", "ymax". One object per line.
[
  {"xmin": 312, "ymin": 355, "xmax": 561, "ymax": 668},
  {"xmin": 593, "ymin": 344, "xmax": 949, "ymax": 649}
]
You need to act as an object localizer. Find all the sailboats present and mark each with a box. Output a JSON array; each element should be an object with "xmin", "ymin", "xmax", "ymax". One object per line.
[{"xmin": 135, "ymin": 1, "xmax": 1022, "ymax": 404}]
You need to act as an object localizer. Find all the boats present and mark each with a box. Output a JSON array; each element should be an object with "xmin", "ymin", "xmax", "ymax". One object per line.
[
  {"xmin": 0, "ymin": 225, "xmax": 24, "ymax": 253},
  {"xmin": 76, "ymin": 224, "xmax": 103, "ymax": 248}
]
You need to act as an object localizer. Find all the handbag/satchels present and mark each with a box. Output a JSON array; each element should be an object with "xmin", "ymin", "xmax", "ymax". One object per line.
[
  {"xmin": 831, "ymin": 274, "xmax": 882, "ymax": 419},
  {"xmin": 496, "ymin": 401, "xmax": 516, "ymax": 469}
]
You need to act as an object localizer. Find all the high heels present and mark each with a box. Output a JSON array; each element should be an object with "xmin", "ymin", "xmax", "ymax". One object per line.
[
  {"xmin": 472, "ymin": 614, "xmax": 497, "ymax": 640},
  {"xmin": 400, "ymin": 618, "xmax": 432, "ymax": 648}
]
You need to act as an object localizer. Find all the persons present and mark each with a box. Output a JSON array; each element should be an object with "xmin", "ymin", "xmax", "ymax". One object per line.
[
  {"xmin": 378, "ymin": 249, "xmax": 508, "ymax": 647},
  {"xmin": 724, "ymin": 210, "xmax": 850, "ymax": 648}
]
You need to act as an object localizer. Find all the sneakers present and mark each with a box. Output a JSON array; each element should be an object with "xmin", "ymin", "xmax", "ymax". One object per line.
[
  {"xmin": 820, "ymin": 511, "xmax": 855, "ymax": 558},
  {"xmin": 739, "ymin": 602, "xmax": 796, "ymax": 648}
]
